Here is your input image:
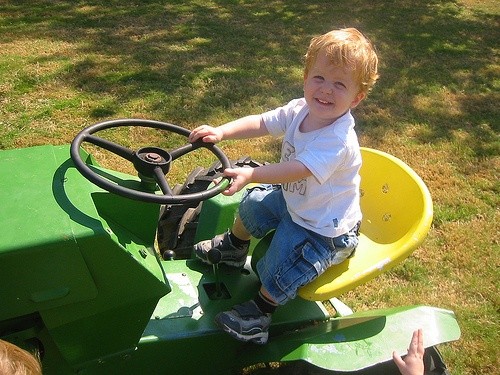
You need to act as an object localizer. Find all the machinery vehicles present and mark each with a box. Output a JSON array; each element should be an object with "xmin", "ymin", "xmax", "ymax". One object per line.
[{"xmin": 0, "ymin": 114, "xmax": 462, "ymax": 374}]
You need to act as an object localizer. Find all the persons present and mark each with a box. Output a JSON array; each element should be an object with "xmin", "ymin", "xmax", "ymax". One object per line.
[{"xmin": 190, "ymin": 27, "xmax": 377, "ymax": 345}]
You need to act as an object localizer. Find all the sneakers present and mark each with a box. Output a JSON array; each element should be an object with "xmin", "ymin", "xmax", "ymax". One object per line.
[
  {"xmin": 216, "ymin": 299, "xmax": 272, "ymax": 345},
  {"xmin": 194, "ymin": 228, "xmax": 249, "ymax": 268}
]
